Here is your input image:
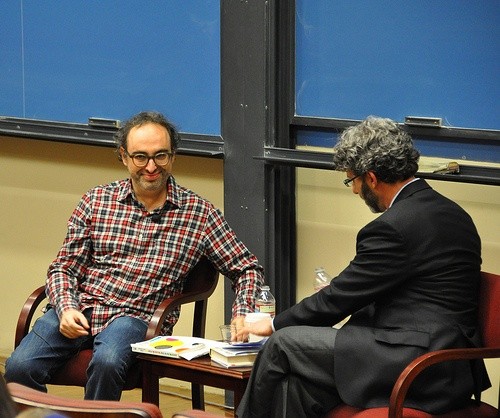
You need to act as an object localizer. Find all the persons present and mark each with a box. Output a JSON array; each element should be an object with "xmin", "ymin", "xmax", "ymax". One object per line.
[
  {"xmin": 236, "ymin": 116, "xmax": 492, "ymax": 418},
  {"xmin": 4, "ymin": 111, "xmax": 266, "ymax": 401}
]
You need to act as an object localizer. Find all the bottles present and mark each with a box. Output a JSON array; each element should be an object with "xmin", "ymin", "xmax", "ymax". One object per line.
[
  {"xmin": 255, "ymin": 286, "xmax": 275, "ymax": 319},
  {"xmin": 313, "ymin": 266, "xmax": 333, "ymax": 292}
]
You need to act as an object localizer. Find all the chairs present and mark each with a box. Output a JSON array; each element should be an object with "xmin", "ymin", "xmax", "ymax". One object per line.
[
  {"xmin": 13, "ymin": 256, "xmax": 218, "ymax": 411},
  {"xmin": 328, "ymin": 272, "xmax": 500, "ymax": 418}
]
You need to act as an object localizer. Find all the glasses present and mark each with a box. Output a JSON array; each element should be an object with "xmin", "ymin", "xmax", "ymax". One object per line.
[
  {"xmin": 125, "ymin": 150, "xmax": 173, "ymax": 166},
  {"xmin": 343, "ymin": 170, "xmax": 367, "ymax": 187}
]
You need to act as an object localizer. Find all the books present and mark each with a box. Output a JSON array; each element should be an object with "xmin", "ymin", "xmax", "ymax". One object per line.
[{"xmin": 130, "ymin": 335, "xmax": 266, "ymax": 369}]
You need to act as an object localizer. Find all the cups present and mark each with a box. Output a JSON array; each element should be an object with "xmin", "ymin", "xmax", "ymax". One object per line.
[{"xmin": 220, "ymin": 325, "xmax": 237, "ymax": 342}]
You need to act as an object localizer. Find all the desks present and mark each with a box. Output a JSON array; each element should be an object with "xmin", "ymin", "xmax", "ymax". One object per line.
[{"xmin": 134, "ymin": 352, "xmax": 252, "ymax": 418}]
